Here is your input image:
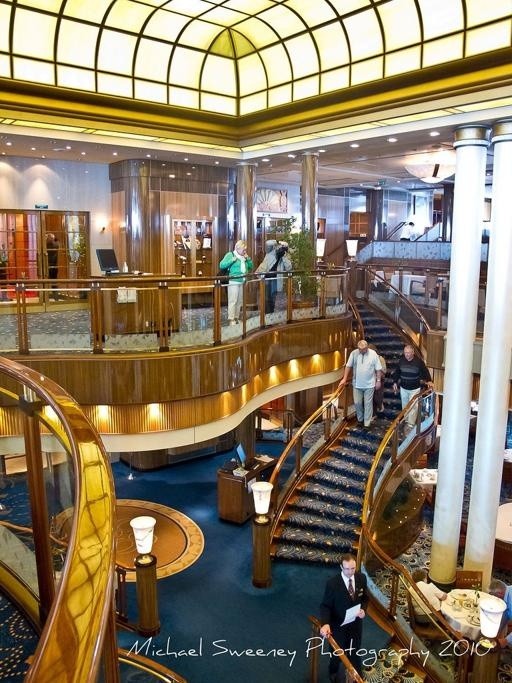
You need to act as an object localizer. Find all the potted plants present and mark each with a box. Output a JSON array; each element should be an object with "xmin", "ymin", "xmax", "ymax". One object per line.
[{"xmin": 274, "ymin": 214, "xmax": 321, "ymax": 321}]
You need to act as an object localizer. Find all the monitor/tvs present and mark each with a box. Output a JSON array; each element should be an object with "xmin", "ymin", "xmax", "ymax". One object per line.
[
  {"xmin": 234, "ymin": 441, "xmax": 248, "ymax": 469},
  {"xmin": 96, "ymin": 248, "xmax": 119, "ymax": 276}
]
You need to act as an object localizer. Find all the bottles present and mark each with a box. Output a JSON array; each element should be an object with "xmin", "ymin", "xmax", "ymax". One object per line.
[{"xmin": 123, "ymin": 262, "xmax": 129, "ymax": 272}]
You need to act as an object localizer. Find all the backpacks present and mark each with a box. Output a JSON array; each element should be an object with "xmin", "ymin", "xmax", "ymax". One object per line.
[{"xmin": 217, "ymin": 269, "xmax": 229, "ymax": 283}]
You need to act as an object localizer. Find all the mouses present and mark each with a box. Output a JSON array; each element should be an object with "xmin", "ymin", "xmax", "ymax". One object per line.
[{"xmin": 256, "ymin": 454, "xmax": 260, "ymax": 458}]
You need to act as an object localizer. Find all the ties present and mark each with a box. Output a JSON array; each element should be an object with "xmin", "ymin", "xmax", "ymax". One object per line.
[
  {"xmin": 504, "ymin": 610, "xmax": 511, "ymax": 621},
  {"xmin": 348, "ymin": 579, "xmax": 355, "ymax": 601}
]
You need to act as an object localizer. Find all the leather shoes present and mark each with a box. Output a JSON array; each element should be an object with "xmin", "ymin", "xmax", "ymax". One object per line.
[
  {"xmin": 361, "ymin": 427, "xmax": 371, "ymax": 432},
  {"xmin": 330, "ymin": 672, "xmax": 337, "ymax": 682},
  {"xmin": 357, "ymin": 422, "xmax": 363, "ymax": 427}
]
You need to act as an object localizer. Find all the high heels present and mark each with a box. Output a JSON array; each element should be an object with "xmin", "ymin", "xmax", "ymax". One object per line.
[
  {"xmin": 371, "ymin": 415, "xmax": 377, "ymax": 424},
  {"xmin": 377, "ymin": 402, "xmax": 385, "ymax": 412}
]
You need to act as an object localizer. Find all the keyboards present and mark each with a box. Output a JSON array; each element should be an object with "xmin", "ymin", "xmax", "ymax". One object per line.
[{"xmin": 247, "ymin": 460, "xmax": 259, "ymax": 469}]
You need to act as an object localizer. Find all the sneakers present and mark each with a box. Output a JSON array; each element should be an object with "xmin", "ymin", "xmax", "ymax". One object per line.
[
  {"xmin": 403, "ymin": 424, "xmax": 412, "ymax": 438},
  {"xmin": 230, "ymin": 318, "xmax": 240, "ymax": 326}
]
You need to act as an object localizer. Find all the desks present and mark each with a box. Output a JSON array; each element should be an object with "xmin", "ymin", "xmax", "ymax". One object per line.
[
  {"xmin": 216, "ymin": 455, "xmax": 278, "ymax": 524},
  {"xmin": 90, "ymin": 273, "xmax": 181, "ymax": 333}
]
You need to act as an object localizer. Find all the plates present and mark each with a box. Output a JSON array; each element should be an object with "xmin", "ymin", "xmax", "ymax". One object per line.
[{"xmin": 448, "ymin": 591, "xmax": 481, "ymax": 626}]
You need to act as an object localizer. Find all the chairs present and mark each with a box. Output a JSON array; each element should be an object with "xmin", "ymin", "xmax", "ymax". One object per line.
[
  {"xmin": 407, "ymin": 569, "xmax": 512, "ymax": 681},
  {"xmin": 365, "ymin": 263, "xmax": 446, "ymax": 295}
]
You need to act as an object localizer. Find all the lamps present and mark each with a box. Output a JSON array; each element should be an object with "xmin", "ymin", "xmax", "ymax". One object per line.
[
  {"xmin": 345, "ymin": 239, "xmax": 358, "ymax": 297},
  {"xmin": 314, "ymin": 238, "xmax": 326, "ymax": 262}
]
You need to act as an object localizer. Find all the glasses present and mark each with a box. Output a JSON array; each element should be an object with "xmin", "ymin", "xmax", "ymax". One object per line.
[{"xmin": 342, "ymin": 565, "xmax": 356, "ymax": 570}]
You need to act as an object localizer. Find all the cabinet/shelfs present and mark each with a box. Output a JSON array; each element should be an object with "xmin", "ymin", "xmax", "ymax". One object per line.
[{"xmin": 173, "ymin": 219, "xmax": 213, "ymax": 277}]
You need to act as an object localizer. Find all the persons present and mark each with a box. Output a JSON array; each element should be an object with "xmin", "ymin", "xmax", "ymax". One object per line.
[
  {"xmin": 339, "ymin": 339, "xmax": 386, "ymax": 434},
  {"xmin": 46, "ymin": 232, "xmax": 60, "ymax": 294},
  {"xmin": 488, "ymin": 579, "xmax": 512, "ymax": 648},
  {"xmin": 408, "ymin": 568, "xmax": 447, "ymax": 629},
  {"xmin": 392, "ymin": 345, "xmax": 434, "ymax": 438},
  {"xmin": 319, "ymin": 553, "xmax": 370, "ymax": 682},
  {"xmin": 400, "ymin": 222, "xmax": 415, "ymax": 240},
  {"xmin": 255, "ymin": 239, "xmax": 292, "ymax": 316},
  {"xmin": 219, "ymin": 240, "xmax": 254, "ymax": 325}
]
